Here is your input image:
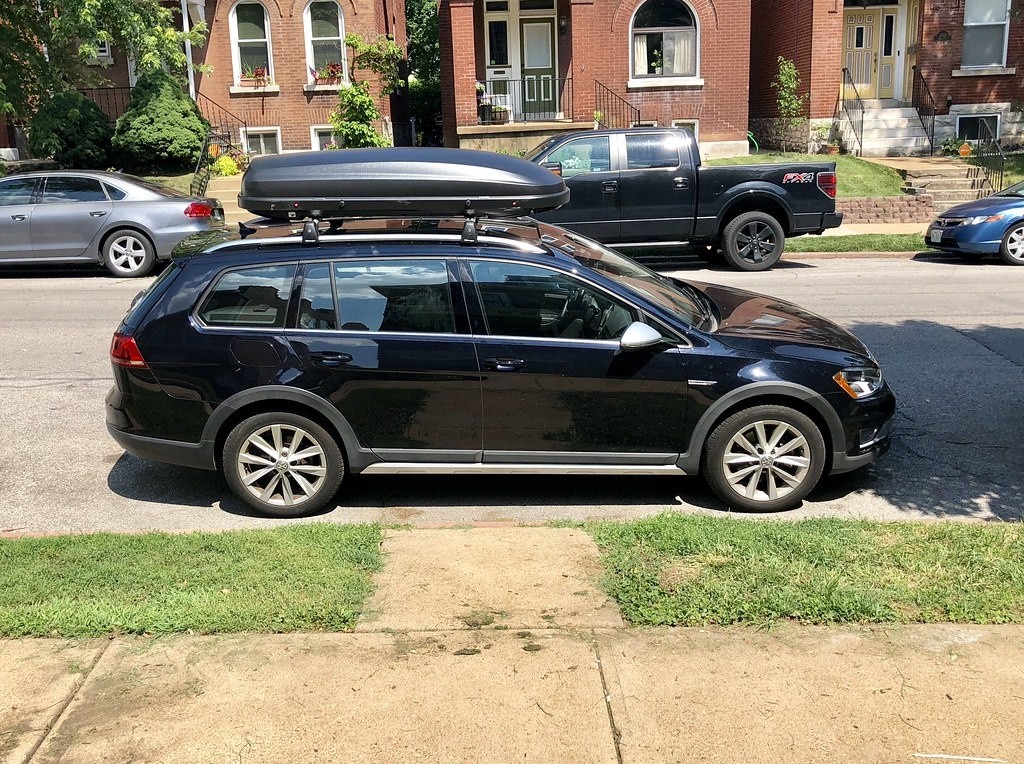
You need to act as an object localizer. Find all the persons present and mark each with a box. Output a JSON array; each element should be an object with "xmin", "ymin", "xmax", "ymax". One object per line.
[
  {"xmin": 4, "ymin": 180, "xmax": 35, "ymax": 204},
  {"xmin": 503, "ymin": 275, "xmax": 585, "ymax": 335}
]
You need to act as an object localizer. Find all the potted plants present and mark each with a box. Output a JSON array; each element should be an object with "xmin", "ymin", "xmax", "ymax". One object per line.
[
  {"xmin": 816, "ymin": 123, "xmax": 830, "ymax": 153},
  {"xmin": 479, "ymin": 103, "xmax": 494, "ymax": 125},
  {"xmin": 828, "ymin": 139, "xmax": 841, "ymax": 155},
  {"xmin": 492, "ymin": 107, "xmax": 507, "ymax": 125}
]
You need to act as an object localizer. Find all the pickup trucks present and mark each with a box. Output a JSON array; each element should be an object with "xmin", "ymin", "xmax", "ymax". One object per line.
[{"xmin": 521, "ymin": 127, "xmax": 843, "ymax": 272}]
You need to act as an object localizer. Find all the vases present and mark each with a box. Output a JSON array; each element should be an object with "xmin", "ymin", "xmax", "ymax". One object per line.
[
  {"xmin": 315, "ymin": 77, "xmax": 341, "ymax": 85},
  {"xmin": 655, "ymin": 66, "xmax": 663, "ymax": 74},
  {"xmin": 476, "ymin": 89, "xmax": 484, "ymax": 96},
  {"xmin": 240, "ymin": 78, "xmax": 268, "ymax": 87}
]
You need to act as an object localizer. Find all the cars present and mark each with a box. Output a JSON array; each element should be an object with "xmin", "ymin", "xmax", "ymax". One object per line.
[
  {"xmin": 0, "ymin": 168, "xmax": 226, "ymax": 278},
  {"xmin": 925, "ymin": 179, "xmax": 1024, "ymax": 265}
]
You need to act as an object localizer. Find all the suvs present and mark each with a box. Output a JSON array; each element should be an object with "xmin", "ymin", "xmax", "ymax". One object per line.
[{"xmin": 104, "ymin": 147, "xmax": 897, "ymax": 518}]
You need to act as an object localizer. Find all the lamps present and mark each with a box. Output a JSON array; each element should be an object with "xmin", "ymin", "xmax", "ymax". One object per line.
[{"xmin": 560, "ymin": 14, "xmax": 567, "ymax": 33}]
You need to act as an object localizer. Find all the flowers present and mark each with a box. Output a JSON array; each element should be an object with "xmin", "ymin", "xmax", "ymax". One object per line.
[
  {"xmin": 240, "ymin": 59, "xmax": 267, "ymax": 82},
  {"xmin": 651, "ymin": 49, "xmax": 663, "ymax": 68},
  {"xmin": 474, "ymin": 80, "xmax": 485, "ymax": 90},
  {"xmin": 308, "ymin": 58, "xmax": 344, "ymax": 80}
]
